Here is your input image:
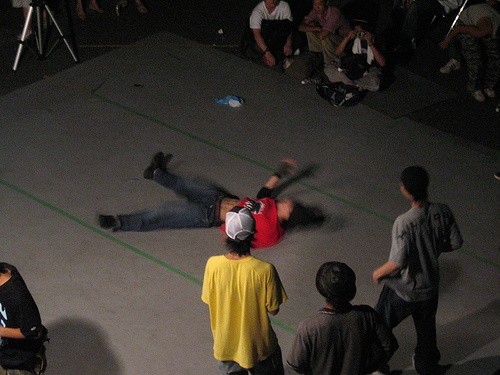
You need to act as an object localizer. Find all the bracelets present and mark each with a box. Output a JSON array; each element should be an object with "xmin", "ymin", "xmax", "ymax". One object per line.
[
  {"xmin": 369, "ymin": 43, "xmax": 375, "ymax": 48},
  {"xmin": 263, "ymin": 48, "xmax": 270, "ymax": 55}
]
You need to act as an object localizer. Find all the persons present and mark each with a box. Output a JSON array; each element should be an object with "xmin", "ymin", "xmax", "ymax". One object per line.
[
  {"xmin": 299, "ymin": 0, "xmax": 353, "ymax": 66},
  {"xmin": 439, "ymin": 4, "xmax": 500, "ymax": 102},
  {"xmin": 286, "ymin": 261, "xmax": 399, "ymax": 375},
  {"xmin": 324, "ymin": 20, "xmax": 385, "ymax": 108},
  {"xmin": 373, "ymin": 166, "xmax": 463, "ymax": 375},
  {"xmin": 250, "ymin": 0, "xmax": 296, "ymax": 66},
  {"xmin": 0, "ymin": 262, "xmax": 41, "ymax": 375},
  {"xmin": 201, "ymin": 205, "xmax": 288, "ymax": 375},
  {"xmin": 376, "ymin": 0, "xmax": 500, "ymax": 66},
  {"xmin": 98, "ymin": 151, "xmax": 299, "ymax": 249},
  {"xmin": 8, "ymin": 0, "xmax": 147, "ymax": 43}
]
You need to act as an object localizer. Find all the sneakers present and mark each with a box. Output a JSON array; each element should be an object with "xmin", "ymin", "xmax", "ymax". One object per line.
[
  {"xmin": 440, "ymin": 58, "xmax": 461, "ymax": 73},
  {"xmin": 143, "ymin": 151, "xmax": 164, "ymax": 180},
  {"xmin": 99, "ymin": 215, "xmax": 115, "ymax": 229},
  {"xmin": 473, "ymin": 89, "xmax": 485, "ymax": 102},
  {"xmin": 485, "ymin": 88, "xmax": 495, "ymax": 97}
]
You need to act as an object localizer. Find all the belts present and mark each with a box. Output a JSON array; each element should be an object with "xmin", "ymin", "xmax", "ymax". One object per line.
[{"xmin": 215, "ymin": 195, "xmax": 224, "ymax": 223}]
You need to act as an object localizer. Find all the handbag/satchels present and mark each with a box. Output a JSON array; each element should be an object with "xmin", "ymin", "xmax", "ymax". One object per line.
[
  {"xmin": 317, "ymin": 75, "xmax": 368, "ymax": 108},
  {"xmin": 283, "ymin": 51, "xmax": 324, "ymax": 81}
]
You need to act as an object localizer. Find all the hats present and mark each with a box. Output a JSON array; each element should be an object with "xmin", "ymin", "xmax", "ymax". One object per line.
[{"xmin": 225, "ymin": 206, "xmax": 256, "ymax": 243}]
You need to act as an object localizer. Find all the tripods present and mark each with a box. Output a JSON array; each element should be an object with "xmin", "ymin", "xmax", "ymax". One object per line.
[{"xmin": 13, "ymin": 0, "xmax": 79, "ymax": 72}]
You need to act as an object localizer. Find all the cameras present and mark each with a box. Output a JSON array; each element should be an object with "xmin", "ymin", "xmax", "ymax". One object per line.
[{"xmin": 355, "ymin": 32, "xmax": 365, "ymax": 39}]
